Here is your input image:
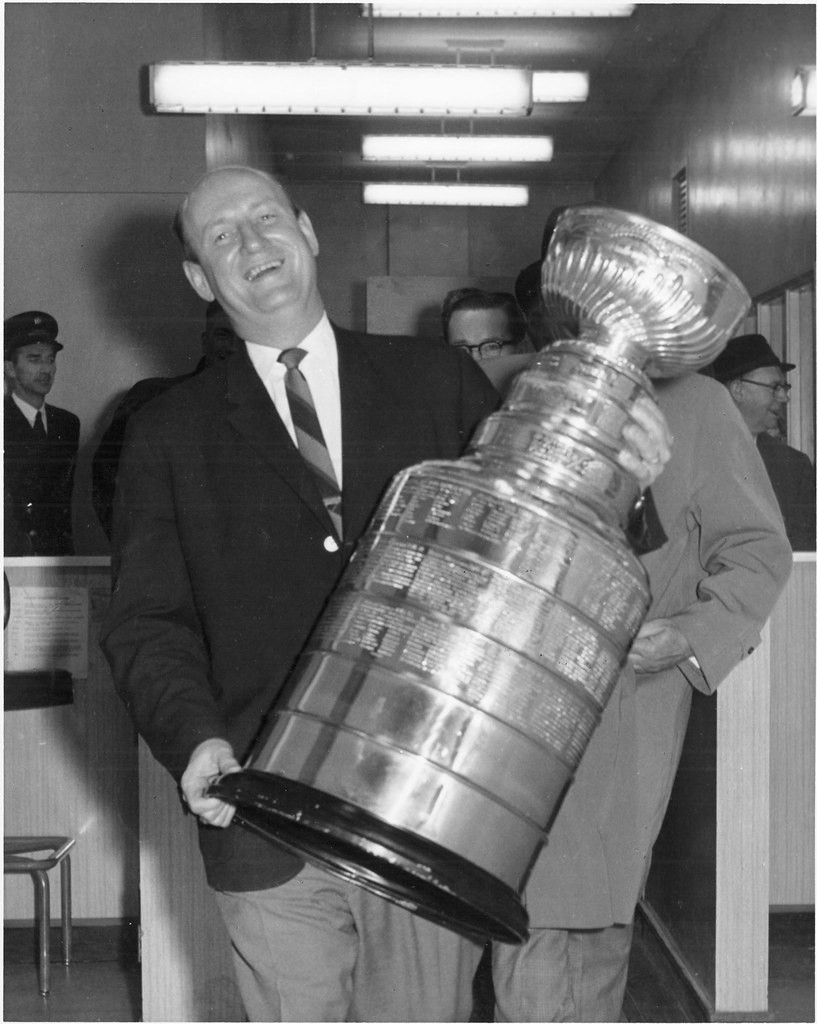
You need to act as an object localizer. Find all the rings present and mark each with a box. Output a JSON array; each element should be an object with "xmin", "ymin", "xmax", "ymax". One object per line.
[{"xmin": 642, "ymin": 448, "xmax": 663, "ymax": 465}]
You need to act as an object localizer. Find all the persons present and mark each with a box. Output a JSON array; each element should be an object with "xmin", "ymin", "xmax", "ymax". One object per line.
[
  {"xmin": 4, "ymin": 310, "xmax": 83, "ymax": 557},
  {"xmin": 440, "ymin": 284, "xmax": 527, "ymax": 361},
  {"xmin": 457, "ymin": 201, "xmax": 795, "ymax": 1024},
  {"xmin": 711, "ymin": 333, "xmax": 816, "ymax": 553},
  {"xmin": 97, "ymin": 164, "xmax": 676, "ymax": 1022},
  {"xmin": 92, "ymin": 299, "xmax": 246, "ymax": 545},
  {"xmin": 514, "ymin": 258, "xmax": 550, "ymax": 353}
]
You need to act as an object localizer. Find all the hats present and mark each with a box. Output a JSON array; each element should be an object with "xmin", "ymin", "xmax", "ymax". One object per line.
[
  {"xmin": 4, "ymin": 311, "xmax": 64, "ymax": 351},
  {"xmin": 714, "ymin": 334, "xmax": 796, "ymax": 382}
]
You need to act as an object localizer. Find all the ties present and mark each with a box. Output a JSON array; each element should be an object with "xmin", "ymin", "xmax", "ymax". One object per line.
[
  {"xmin": 277, "ymin": 347, "xmax": 343, "ymax": 543},
  {"xmin": 32, "ymin": 411, "xmax": 47, "ymax": 441}
]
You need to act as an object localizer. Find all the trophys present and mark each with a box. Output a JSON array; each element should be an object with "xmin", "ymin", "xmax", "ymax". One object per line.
[{"xmin": 199, "ymin": 207, "xmax": 754, "ymax": 946}]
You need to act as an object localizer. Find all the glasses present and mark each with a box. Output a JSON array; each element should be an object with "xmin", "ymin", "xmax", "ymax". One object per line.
[
  {"xmin": 453, "ymin": 336, "xmax": 518, "ymax": 360},
  {"xmin": 739, "ymin": 379, "xmax": 791, "ymax": 397}
]
